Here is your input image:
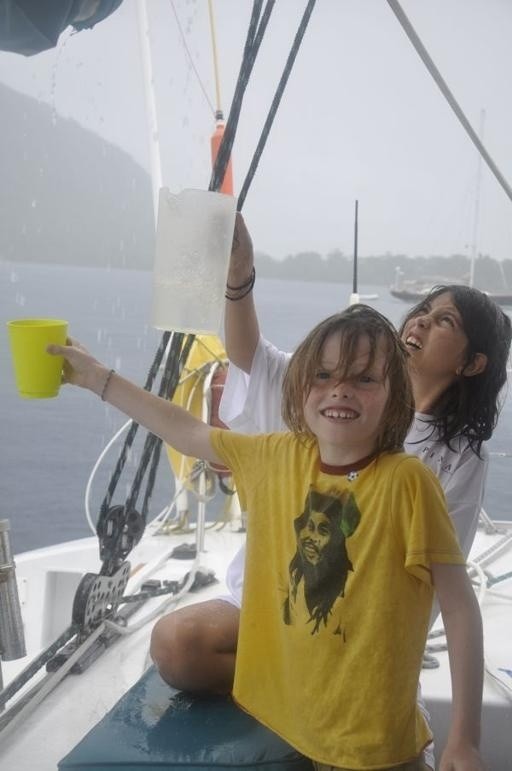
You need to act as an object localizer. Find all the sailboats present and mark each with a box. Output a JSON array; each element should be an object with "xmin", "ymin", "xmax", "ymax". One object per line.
[{"xmin": 385, "ymin": 110, "xmax": 512, "ymax": 307}]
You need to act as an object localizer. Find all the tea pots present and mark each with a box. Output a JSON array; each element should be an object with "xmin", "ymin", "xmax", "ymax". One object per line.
[{"xmin": 148, "ymin": 186, "xmax": 240, "ymax": 336}]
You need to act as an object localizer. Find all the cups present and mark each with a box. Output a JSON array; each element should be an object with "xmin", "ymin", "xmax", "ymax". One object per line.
[{"xmin": 5, "ymin": 317, "xmax": 70, "ymax": 399}]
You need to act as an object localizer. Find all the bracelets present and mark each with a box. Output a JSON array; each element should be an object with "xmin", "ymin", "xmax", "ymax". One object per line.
[
  {"xmin": 223, "ymin": 265, "xmax": 257, "ymax": 300},
  {"xmin": 95, "ymin": 364, "xmax": 116, "ymax": 401}
]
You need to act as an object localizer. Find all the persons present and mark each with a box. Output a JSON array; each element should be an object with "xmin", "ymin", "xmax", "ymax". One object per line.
[
  {"xmin": 149, "ymin": 208, "xmax": 511, "ymax": 696},
  {"xmin": 44, "ymin": 302, "xmax": 489, "ymax": 771},
  {"xmin": 281, "ymin": 481, "xmax": 362, "ymax": 632}
]
